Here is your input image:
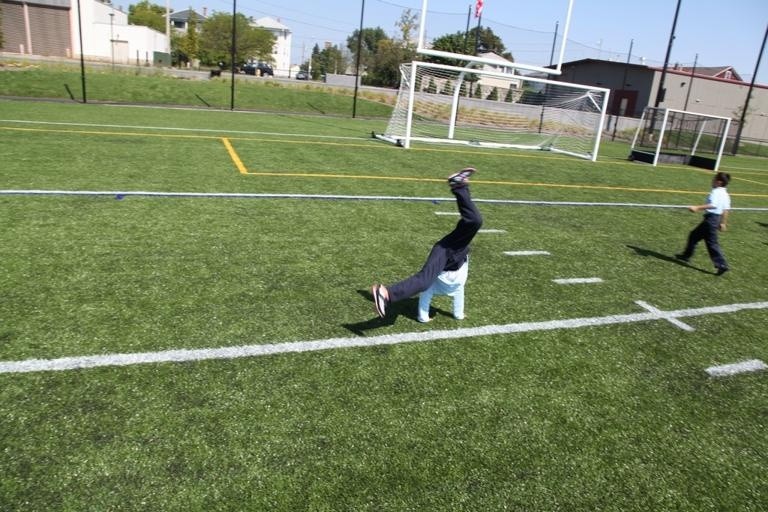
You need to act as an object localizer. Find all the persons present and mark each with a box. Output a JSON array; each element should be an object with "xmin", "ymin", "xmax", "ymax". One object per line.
[
  {"xmin": 372, "ymin": 169, "xmax": 482, "ymax": 324},
  {"xmin": 675, "ymin": 172, "xmax": 732, "ymax": 276}
]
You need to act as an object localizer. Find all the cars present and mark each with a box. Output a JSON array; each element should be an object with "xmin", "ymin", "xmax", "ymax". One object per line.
[
  {"xmin": 296, "ymin": 71, "xmax": 306, "ymax": 80},
  {"xmin": 241, "ymin": 61, "xmax": 274, "ymax": 78}
]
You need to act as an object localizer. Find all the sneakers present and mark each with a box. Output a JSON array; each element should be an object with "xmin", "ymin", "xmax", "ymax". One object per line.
[
  {"xmin": 448, "ymin": 168, "xmax": 476, "ymax": 184},
  {"xmin": 714, "ymin": 268, "xmax": 729, "ymax": 275},
  {"xmin": 372, "ymin": 284, "xmax": 386, "ymax": 318},
  {"xmin": 676, "ymin": 255, "xmax": 689, "ymax": 262}
]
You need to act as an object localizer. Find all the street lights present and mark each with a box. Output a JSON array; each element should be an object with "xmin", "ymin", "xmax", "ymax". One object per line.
[{"xmin": 108, "ymin": 8, "xmax": 116, "ymax": 72}]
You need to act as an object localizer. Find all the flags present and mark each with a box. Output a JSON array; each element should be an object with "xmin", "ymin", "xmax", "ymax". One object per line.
[{"xmin": 473, "ymin": 0, "xmax": 486, "ymax": 20}]
